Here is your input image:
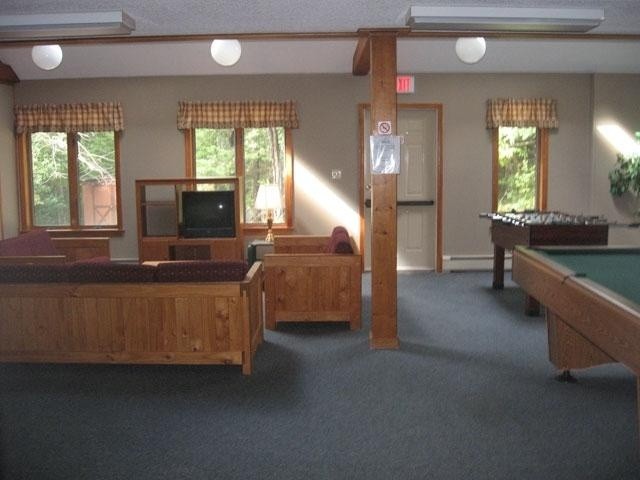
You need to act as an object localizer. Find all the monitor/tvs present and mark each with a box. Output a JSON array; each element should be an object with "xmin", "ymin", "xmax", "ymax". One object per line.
[{"xmin": 182, "ymin": 191, "xmax": 236, "ymax": 239}]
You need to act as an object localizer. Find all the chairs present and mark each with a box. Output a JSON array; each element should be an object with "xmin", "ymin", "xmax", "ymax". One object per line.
[
  {"xmin": 263, "ymin": 227, "xmax": 363, "ymax": 333},
  {"xmin": 0, "ymin": 229, "xmax": 111, "ymax": 261}
]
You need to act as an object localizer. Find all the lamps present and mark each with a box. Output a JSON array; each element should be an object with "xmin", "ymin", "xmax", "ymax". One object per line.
[
  {"xmin": 456, "ymin": 38, "xmax": 487, "ymax": 65},
  {"xmin": 255, "ymin": 184, "xmax": 281, "ymax": 242},
  {"xmin": 406, "ymin": 7, "xmax": 605, "ymax": 33},
  {"xmin": 32, "ymin": 45, "xmax": 63, "ymax": 70},
  {"xmin": 210, "ymin": 39, "xmax": 243, "ymax": 66},
  {"xmin": 0, "ymin": 11, "xmax": 136, "ymax": 43}
]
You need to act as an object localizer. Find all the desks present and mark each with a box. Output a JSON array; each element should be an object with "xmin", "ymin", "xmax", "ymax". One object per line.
[{"xmin": 510, "ymin": 244, "xmax": 640, "ymax": 381}]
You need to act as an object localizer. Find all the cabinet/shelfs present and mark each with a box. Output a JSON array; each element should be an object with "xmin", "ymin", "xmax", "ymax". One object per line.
[{"xmin": 136, "ymin": 177, "xmax": 244, "ymax": 261}]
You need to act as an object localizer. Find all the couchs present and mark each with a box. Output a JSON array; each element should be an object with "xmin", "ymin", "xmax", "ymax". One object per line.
[{"xmin": 0, "ymin": 260, "xmax": 264, "ymax": 378}]
